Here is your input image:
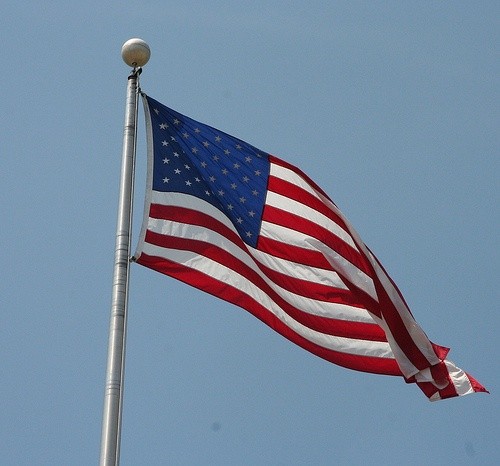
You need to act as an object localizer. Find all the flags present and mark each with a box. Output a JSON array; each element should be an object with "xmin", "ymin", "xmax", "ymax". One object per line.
[{"xmin": 130, "ymin": 91, "xmax": 489, "ymax": 401}]
